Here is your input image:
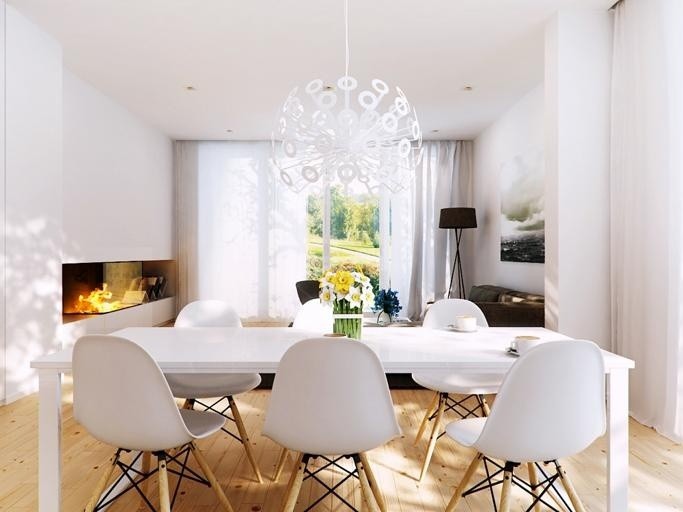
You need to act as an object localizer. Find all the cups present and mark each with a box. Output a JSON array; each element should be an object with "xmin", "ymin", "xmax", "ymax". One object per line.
[
  {"xmin": 510, "ymin": 335, "xmax": 542, "ymax": 354},
  {"xmin": 454, "ymin": 315, "xmax": 477, "ymax": 330},
  {"xmin": 321, "ymin": 333, "xmax": 347, "ymax": 339}
]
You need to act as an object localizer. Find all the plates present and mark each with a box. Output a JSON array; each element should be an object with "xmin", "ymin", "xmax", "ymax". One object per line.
[
  {"xmin": 444, "ymin": 325, "xmax": 479, "ymax": 332},
  {"xmin": 506, "ymin": 346, "xmax": 525, "ymax": 356}
]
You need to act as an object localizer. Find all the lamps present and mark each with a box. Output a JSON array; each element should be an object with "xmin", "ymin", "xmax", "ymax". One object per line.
[
  {"xmin": 270, "ymin": 0, "xmax": 425, "ymax": 201},
  {"xmin": 439, "ymin": 207, "xmax": 478, "ymax": 300}
]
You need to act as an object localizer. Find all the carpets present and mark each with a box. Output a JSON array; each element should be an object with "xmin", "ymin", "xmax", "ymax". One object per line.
[{"xmin": 252, "ymin": 374, "xmax": 432, "ymax": 391}]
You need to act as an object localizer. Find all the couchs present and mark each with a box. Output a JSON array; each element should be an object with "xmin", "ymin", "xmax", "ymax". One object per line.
[{"xmin": 425, "ymin": 284, "xmax": 547, "ymax": 326}]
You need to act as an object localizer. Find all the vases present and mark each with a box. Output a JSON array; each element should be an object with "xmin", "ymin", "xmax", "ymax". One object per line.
[{"xmin": 332, "ymin": 300, "xmax": 362, "ymax": 340}]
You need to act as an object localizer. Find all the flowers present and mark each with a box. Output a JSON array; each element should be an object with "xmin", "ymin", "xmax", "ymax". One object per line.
[
  {"xmin": 318, "ymin": 265, "xmax": 377, "ymax": 336},
  {"xmin": 375, "ymin": 288, "xmax": 403, "ymax": 318}
]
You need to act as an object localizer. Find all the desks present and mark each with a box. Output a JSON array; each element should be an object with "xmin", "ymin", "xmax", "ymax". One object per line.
[{"xmin": 30, "ymin": 326, "xmax": 636, "ymax": 512}]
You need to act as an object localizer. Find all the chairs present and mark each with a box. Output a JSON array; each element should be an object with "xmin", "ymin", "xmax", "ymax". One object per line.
[
  {"xmin": 274, "ymin": 297, "xmax": 333, "ymax": 480},
  {"xmin": 411, "ymin": 299, "xmax": 491, "ymax": 482},
  {"xmin": 71, "ymin": 335, "xmax": 234, "ymax": 512},
  {"xmin": 262, "ymin": 338, "xmax": 402, "ymax": 512},
  {"xmin": 440, "ymin": 340, "xmax": 607, "ymax": 512},
  {"xmin": 295, "ymin": 279, "xmax": 322, "ymax": 304},
  {"xmin": 163, "ymin": 300, "xmax": 261, "ymax": 484}
]
